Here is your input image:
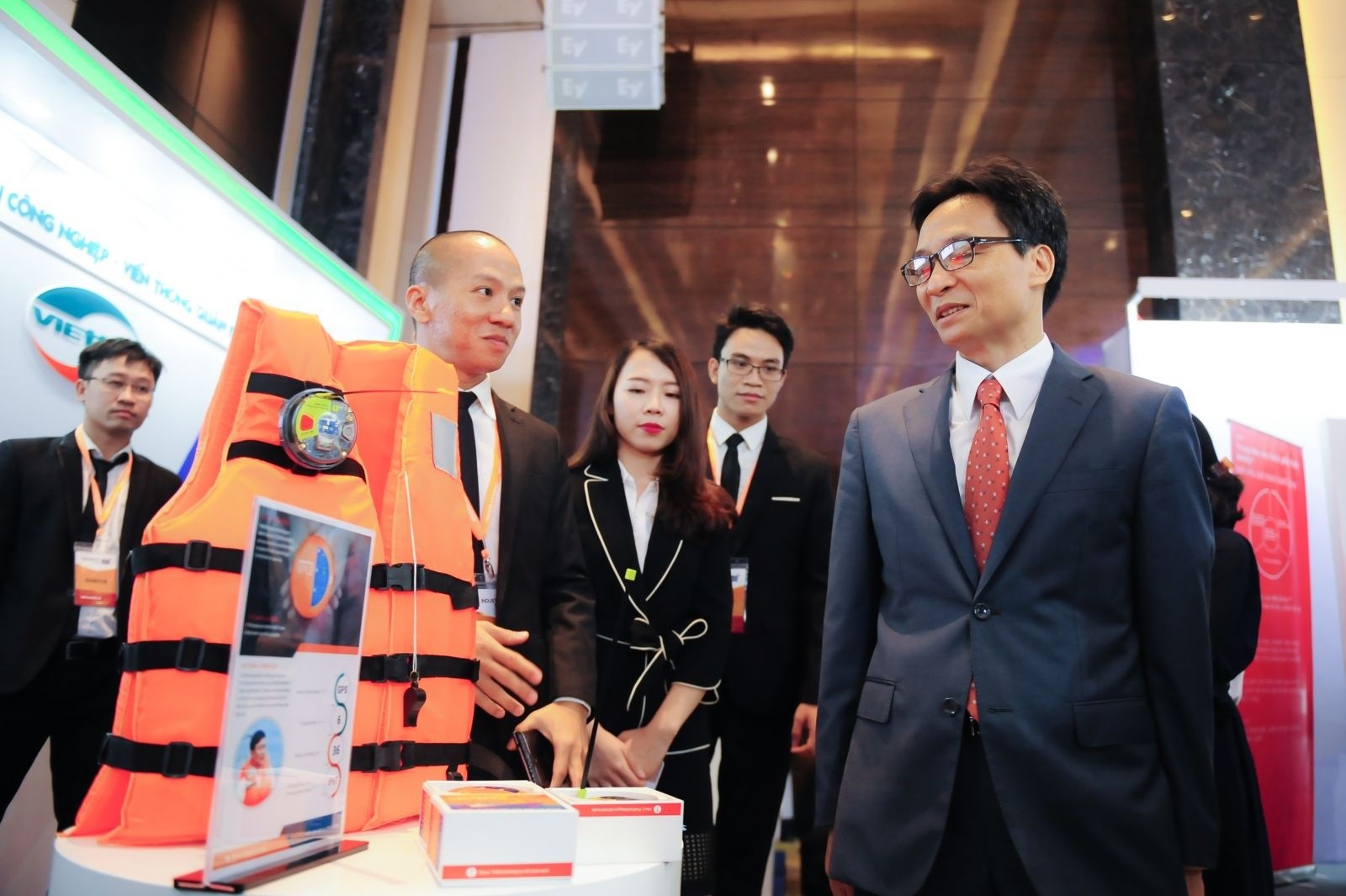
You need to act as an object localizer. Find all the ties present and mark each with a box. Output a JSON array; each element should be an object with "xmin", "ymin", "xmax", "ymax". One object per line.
[
  {"xmin": 453, "ymin": 390, "xmax": 485, "ymax": 586},
  {"xmin": 69, "ymin": 452, "xmax": 129, "ymax": 636},
  {"xmin": 720, "ymin": 436, "xmax": 744, "ymax": 506},
  {"xmin": 967, "ymin": 377, "xmax": 1007, "ymax": 719}
]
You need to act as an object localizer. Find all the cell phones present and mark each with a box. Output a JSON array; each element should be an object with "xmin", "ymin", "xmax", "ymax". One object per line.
[{"xmin": 512, "ymin": 729, "xmax": 554, "ymax": 788}]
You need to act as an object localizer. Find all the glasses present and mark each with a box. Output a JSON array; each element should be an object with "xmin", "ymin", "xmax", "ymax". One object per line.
[
  {"xmin": 900, "ymin": 238, "xmax": 1038, "ymax": 287},
  {"xmin": 717, "ymin": 354, "xmax": 785, "ymax": 381},
  {"xmin": 83, "ymin": 374, "xmax": 152, "ymax": 395}
]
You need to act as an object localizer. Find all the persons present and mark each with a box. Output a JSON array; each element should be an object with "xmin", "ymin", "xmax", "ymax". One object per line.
[
  {"xmin": 680, "ymin": 302, "xmax": 832, "ymax": 895},
  {"xmin": 1185, "ymin": 415, "xmax": 1277, "ymax": 896},
  {"xmin": 559, "ymin": 334, "xmax": 739, "ymax": 896},
  {"xmin": 237, "ymin": 728, "xmax": 274, "ymax": 805},
  {"xmin": 808, "ymin": 154, "xmax": 1225, "ymax": 896},
  {"xmin": 397, "ymin": 228, "xmax": 607, "ymax": 796},
  {"xmin": 0, "ymin": 341, "xmax": 184, "ymax": 833}
]
